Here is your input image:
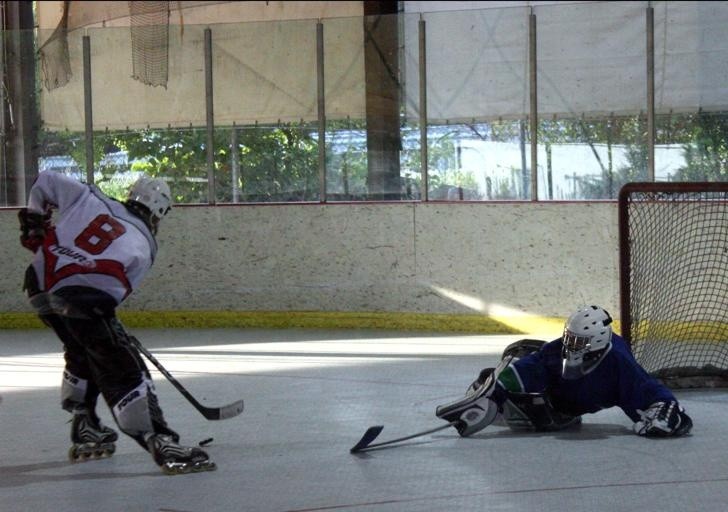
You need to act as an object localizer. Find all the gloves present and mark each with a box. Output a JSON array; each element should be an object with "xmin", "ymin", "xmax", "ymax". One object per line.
[{"xmin": 18, "ymin": 208, "xmax": 52, "ymax": 254}]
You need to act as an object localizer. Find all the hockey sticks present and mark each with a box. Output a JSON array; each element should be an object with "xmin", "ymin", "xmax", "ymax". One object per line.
[
  {"xmin": 29, "ymin": 228, "xmax": 243, "ymax": 420},
  {"xmin": 349, "ymin": 420, "xmax": 459, "ymax": 453}
]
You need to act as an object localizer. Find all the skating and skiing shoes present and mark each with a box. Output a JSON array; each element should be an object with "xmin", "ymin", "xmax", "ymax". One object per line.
[
  {"xmin": 65, "ymin": 407, "xmax": 121, "ymax": 465},
  {"xmin": 147, "ymin": 431, "xmax": 217, "ymax": 475}
]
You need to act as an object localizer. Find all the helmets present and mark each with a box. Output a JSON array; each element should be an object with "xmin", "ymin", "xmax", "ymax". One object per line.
[
  {"xmin": 126, "ymin": 177, "xmax": 174, "ymax": 228},
  {"xmin": 559, "ymin": 306, "xmax": 612, "ymax": 382}
]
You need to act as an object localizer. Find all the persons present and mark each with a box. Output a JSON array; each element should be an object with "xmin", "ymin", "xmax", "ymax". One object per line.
[
  {"xmin": 16, "ymin": 167, "xmax": 211, "ymax": 466},
  {"xmin": 433, "ymin": 301, "xmax": 694, "ymax": 439}
]
